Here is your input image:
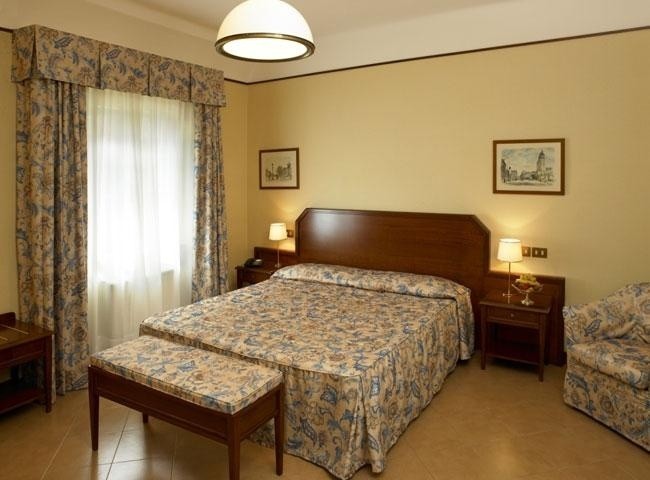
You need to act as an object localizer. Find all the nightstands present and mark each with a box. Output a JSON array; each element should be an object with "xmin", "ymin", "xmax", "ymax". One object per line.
[
  {"xmin": 480, "ymin": 291, "xmax": 554, "ymax": 382},
  {"xmin": 235, "ymin": 263, "xmax": 275, "ymax": 289}
]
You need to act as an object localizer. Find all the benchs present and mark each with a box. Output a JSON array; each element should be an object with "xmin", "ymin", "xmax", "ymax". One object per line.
[{"xmin": 87, "ymin": 336, "xmax": 286, "ymax": 480}]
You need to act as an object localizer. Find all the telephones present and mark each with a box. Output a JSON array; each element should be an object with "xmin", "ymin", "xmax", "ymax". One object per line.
[{"xmin": 244, "ymin": 258, "xmax": 263, "ymax": 267}]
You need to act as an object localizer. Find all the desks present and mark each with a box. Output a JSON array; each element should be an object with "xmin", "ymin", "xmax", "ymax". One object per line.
[{"xmin": 1, "ymin": 312, "xmax": 53, "ymax": 417}]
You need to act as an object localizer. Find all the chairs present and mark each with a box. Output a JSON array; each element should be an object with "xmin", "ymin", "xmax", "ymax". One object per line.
[{"xmin": 562, "ymin": 282, "xmax": 650, "ymax": 452}]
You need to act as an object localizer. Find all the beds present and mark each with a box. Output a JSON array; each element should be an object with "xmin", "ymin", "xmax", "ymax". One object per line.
[{"xmin": 137, "ymin": 207, "xmax": 492, "ymax": 476}]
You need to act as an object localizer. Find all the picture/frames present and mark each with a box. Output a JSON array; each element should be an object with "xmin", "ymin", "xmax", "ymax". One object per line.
[
  {"xmin": 491, "ymin": 137, "xmax": 565, "ymax": 197},
  {"xmin": 258, "ymin": 147, "xmax": 299, "ymax": 190}
]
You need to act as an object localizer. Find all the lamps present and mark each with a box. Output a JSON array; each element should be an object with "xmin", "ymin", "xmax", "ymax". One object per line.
[
  {"xmin": 496, "ymin": 239, "xmax": 524, "ymax": 296},
  {"xmin": 268, "ymin": 222, "xmax": 288, "ymax": 269},
  {"xmin": 213, "ymin": 0, "xmax": 315, "ymax": 64}
]
